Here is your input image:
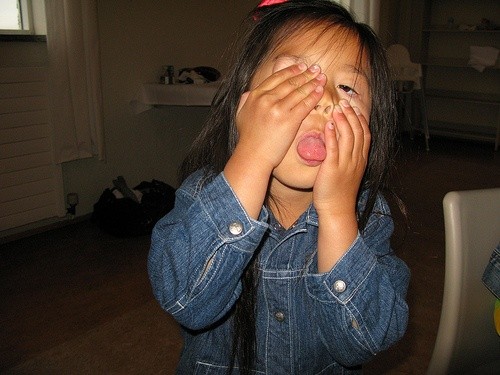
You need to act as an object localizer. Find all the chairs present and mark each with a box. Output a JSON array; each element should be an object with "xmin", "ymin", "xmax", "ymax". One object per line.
[{"xmin": 426, "ymin": 188, "xmax": 500, "ymax": 375}]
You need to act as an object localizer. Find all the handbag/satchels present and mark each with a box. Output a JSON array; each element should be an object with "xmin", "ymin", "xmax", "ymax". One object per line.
[{"xmin": 93, "ymin": 177, "xmax": 177, "ymax": 238}]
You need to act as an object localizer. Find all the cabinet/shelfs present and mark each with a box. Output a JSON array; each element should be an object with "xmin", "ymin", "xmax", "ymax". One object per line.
[{"xmin": 409, "ymin": 0, "xmax": 500, "ymax": 150}]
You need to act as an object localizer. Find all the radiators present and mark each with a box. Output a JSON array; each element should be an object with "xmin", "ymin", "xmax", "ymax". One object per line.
[{"xmin": 0, "ymin": 67, "xmax": 66, "ymax": 232}]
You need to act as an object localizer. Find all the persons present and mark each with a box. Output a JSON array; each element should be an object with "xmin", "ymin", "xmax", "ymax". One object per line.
[{"xmin": 146, "ymin": 1, "xmax": 416, "ymax": 375}]
